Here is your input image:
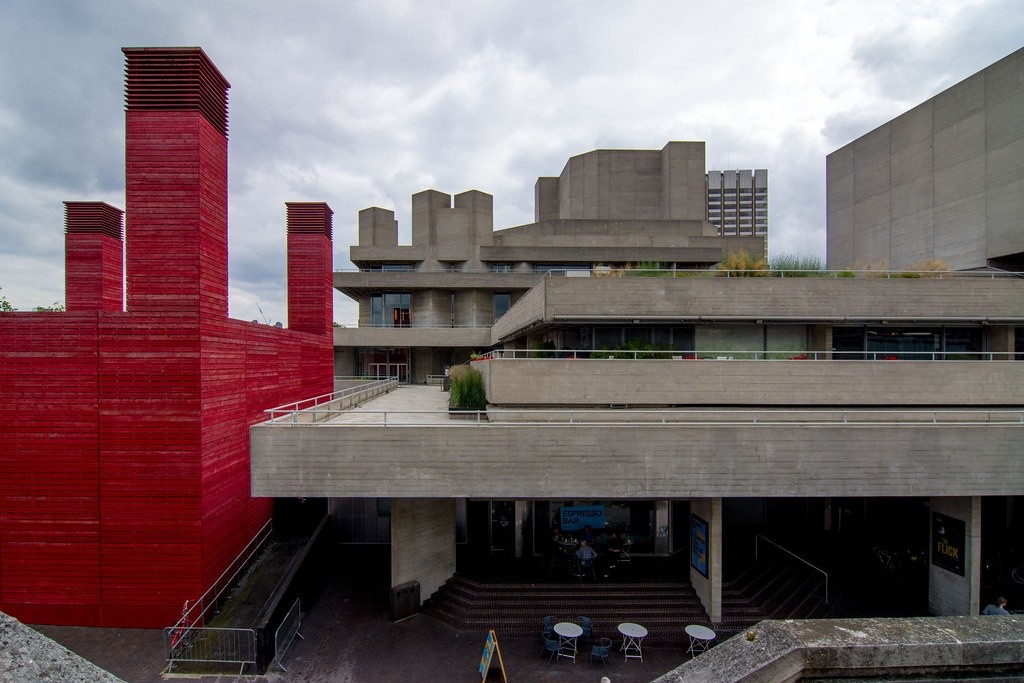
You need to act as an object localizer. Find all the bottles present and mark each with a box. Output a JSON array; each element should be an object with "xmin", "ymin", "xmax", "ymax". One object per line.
[{"xmin": 570, "ymin": 536, "xmax": 573, "ymax": 541}]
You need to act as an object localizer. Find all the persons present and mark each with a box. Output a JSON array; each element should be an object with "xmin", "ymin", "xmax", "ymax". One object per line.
[
  {"xmin": 581, "ymin": 525, "xmax": 595, "ymax": 545},
  {"xmin": 548, "ymin": 528, "xmax": 566, "ymax": 555},
  {"xmin": 982, "ymin": 597, "xmax": 1011, "ymax": 615},
  {"xmin": 573, "ymin": 540, "xmax": 597, "ymax": 576},
  {"xmin": 603, "ymin": 532, "xmax": 625, "ymax": 577}
]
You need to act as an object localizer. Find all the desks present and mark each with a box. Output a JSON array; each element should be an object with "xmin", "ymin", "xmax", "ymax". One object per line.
[
  {"xmin": 554, "ymin": 621, "xmax": 584, "ymax": 664},
  {"xmin": 685, "ymin": 624, "xmax": 717, "ymax": 659},
  {"xmin": 615, "ymin": 538, "xmax": 634, "ymax": 567},
  {"xmin": 617, "ymin": 623, "xmax": 648, "ymax": 664},
  {"xmin": 555, "ymin": 538, "xmax": 580, "ymax": 563}
]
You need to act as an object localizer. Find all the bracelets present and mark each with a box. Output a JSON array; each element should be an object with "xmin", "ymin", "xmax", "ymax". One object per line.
[{"xmin": 591, "ymin": 543, "xmax": 592, "ymax": 544}]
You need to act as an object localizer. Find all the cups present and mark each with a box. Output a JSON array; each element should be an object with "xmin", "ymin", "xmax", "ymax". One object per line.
[{"xmin": 627, "ymin": 539, "xmax": 630, "ymax": 543}]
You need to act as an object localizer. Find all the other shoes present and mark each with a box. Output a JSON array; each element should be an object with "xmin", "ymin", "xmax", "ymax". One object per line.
[
  {"xmin": 604, "ymin": 573, "xmax": 610, "ymax": 577},
  {"xmin": 577, "ymin": 574, "xmax": 586, "ymax": 576},
  {"xmin": 610, "ymin": 565, "xmax": 616, "ymax": 569}
]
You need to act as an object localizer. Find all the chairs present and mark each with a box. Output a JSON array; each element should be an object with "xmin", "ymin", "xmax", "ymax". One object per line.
[
  {"xmin": 590, "ymin": 637, "xmax": 614, "ymax": 674},
  {"xmin": 541, "ymin": 615, "xmax": 563, "ymax": 642},
  {"xmin": 579, "ymin": 616, "xmax": 594, "ymax": 643},
  {"xmin": 539, "ymin": 631, "xmax": 564, "ymax": 665},
  {"xmin": 574, "ymin": 557, "xmax": 596, "ymax": 581}
]
